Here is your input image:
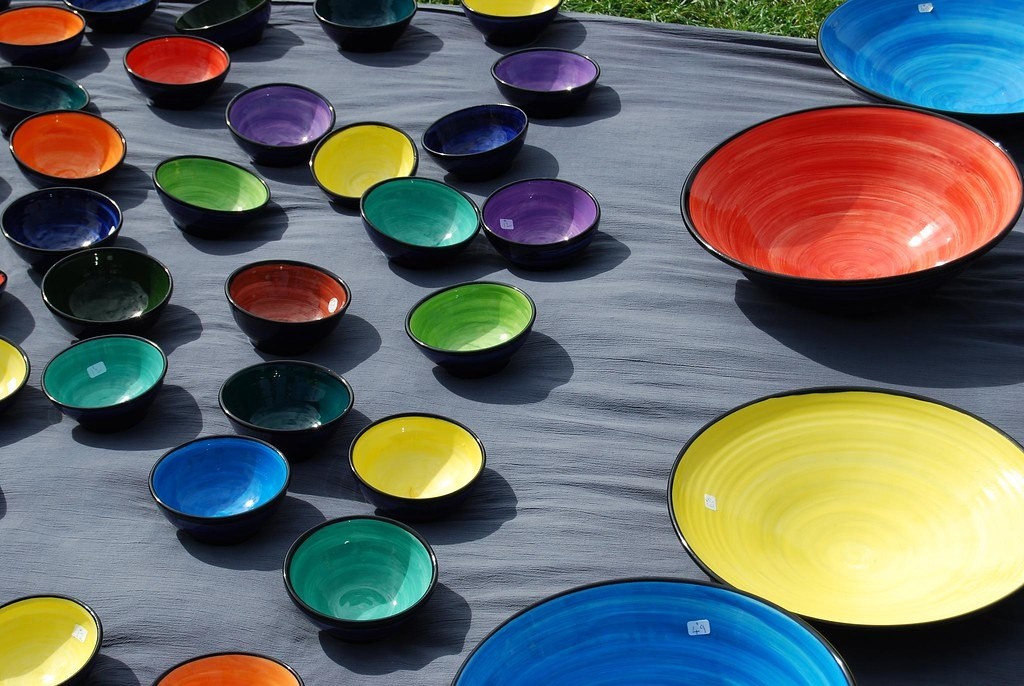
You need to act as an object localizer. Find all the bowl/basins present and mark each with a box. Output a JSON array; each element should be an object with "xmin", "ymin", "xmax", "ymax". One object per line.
[
  {"xmin": 0, "ymin": 593, "xmax": 103, "ymax": 686},
  {"xmin": 123, "ymin": 34, "xmax": 231, "ymax": 108},
  {"xmin": 816, "ymin": 0, "xmax": 1024, "ymax": 116},
  {"xmin": 0, "ymin": 270, "xmax": 32, "ymax": 401},
  {"xmin": 404, "ymin": 282, "xmax": 536, "ymax": 371},
  {"xmin": 490, "ymin": 47, "xmax": 600, "ymax": 110},
  {"xmin": 152, "ymin": 155, "xmax": 271, "ymax": 235},
  {"xmin": 218, "ymin": 360, "xmax": 355, "ymax": 446},
  {"xmin": 348, "ymin": 412, "xmax": 486, "ymax": 515},
  {"xmin": 64, "ymin": 0, "xmax": 565, "ymax": 52},
  {"xmin": 667, "ymin": 386, "xmax": 1024, "ymax": 628},
  {"xmin": 10, "ymin": 110, "xmax": 127, "ymax": 190},
  {"xmin": 0, "ymin": 187, "xmax": 123, "ymax": 270},
  {"xmin": 147, "ymin": 434, "xmax": 291, "ymax": 536},
  {"xmin": 151, "ymin": 651, "xmax": 305, "ymax": 686},
  {"xmin": 40, "ymin": 247, "xmax": 174, "ymax": 326},
  {"xmin": 0, "ymin": 5, "xmax": 90, "ymax": 134},
  {"xmin": 680, "ymin": 104, "xmax": 1024, "ymax": 293},
  {"xmin": 350, "ymin": 176, "xmax": 603, "ymax": 261},
  {"xmin": 450, "ymin": 576, "xmax": 857, "ymax": 686},
  {"xmin": 42, "ymin": 334, "xmax": 168, "ymax": 424},
  {"xmin": 283, "ymin": 515, "xmax": 438, "ymax": 628},
  {"xmin": 221, "ymin": 75, "xmax": 532, "ymax": 200},
  {"xmin": 224, "ymin": 260, "xmax": 351, "ymax": 355}
]
